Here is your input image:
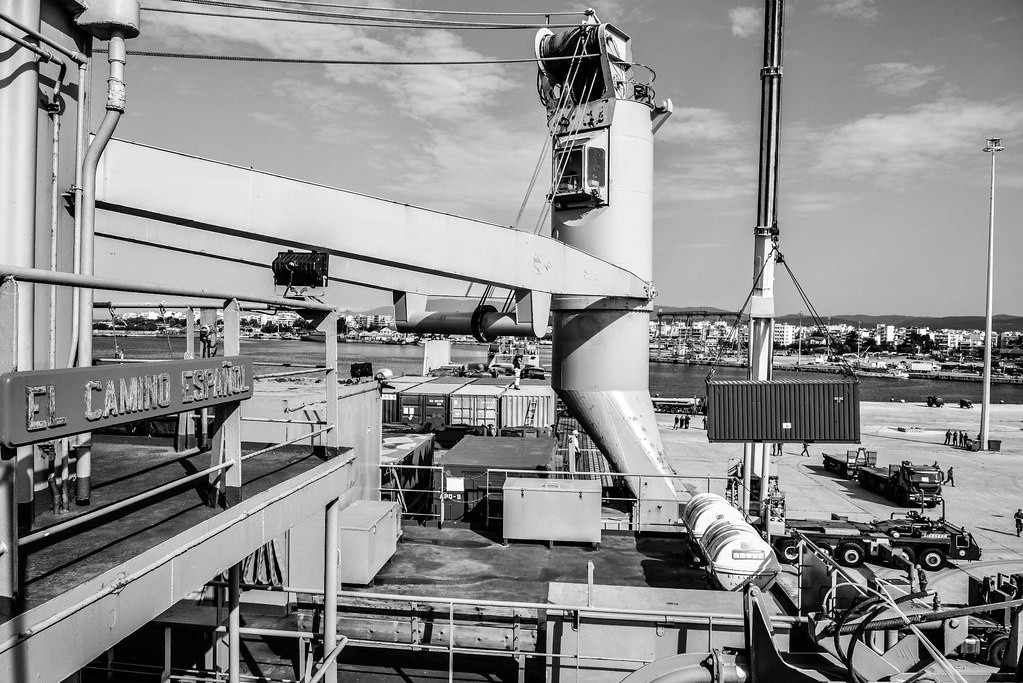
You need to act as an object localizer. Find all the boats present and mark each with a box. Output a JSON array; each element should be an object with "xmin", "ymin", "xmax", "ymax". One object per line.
[
  {"xmin": 649, "ymin": 351, "xmax": 782, "ymax": 369},
  {"xmin": 799, "ymin": 357, "xmax": 1023, "ymax": 383},
  {"xmin": 249, "ymin": 332, "xmax": 346, "ymax": 343}
]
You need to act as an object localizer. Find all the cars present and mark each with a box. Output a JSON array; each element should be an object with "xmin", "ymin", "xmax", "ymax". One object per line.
[{"xmin": 431, "ymin": 363, "xmax": 545, "ymax": 380}]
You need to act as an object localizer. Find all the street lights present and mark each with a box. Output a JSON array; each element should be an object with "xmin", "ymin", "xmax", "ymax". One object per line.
[
  {"xmin": 826, "ymin": 317, "xmax": 831, "ymax": 355},
  {"xmin": 798, "ymin": 311, "xmax": 803, "ymax": 366},
  {"xmin": 857, "ymin": 321, "xmax": 860, "ymax": 357}
]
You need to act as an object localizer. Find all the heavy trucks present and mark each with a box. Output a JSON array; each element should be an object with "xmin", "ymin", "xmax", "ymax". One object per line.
[
  {"xmin": 822, "ymin": 448, "xmax": 944, "ymax": 508},
  {"xmin": 650, "ymin": 398, "xmax": 701, "ymax": 414}
]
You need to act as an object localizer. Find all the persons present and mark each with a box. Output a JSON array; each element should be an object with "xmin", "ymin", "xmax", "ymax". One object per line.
[
  {"xmin": 952, "ymin": 430, "xmax": 957, "ymax": 446},
  {"xmin": 963, "ymin": 433, "xmax": 968, "ymax": 448},
  {"xmin": 801, "ymin": 442, "xmax": 810, "ymax": 457},
  {"xmin": 1014, "ymin": 509, "xmax": 1023, "ymax": 537},
  {"xmin": 928, "ymin": 395, "xmax": 935, "ymax": 402},
  {"xmin": 569, "ymin": 430, "xmax": 581, "ymax": 466},
  {"xmin": 680, "ymin": 415, "xmax": 684, "ymax": 428},
  {"xmin": 773, "ymin": 442, "xmax": 776, "ymax": 456},
  {"xmin": 512, "ymin": 348, "xmax": 523, "ymax": 391},
  {"xmin": 685, "ymin": 415, "xmax": 690, "ymax": 428},
  {"xmin": 958, "ymin": 431, "xmax": 963, "ymax": 447},
  {"xmin": 932, "ymin": 461, "xmax": 940, "ymax": 471},
  {"xmin": 943, "ymin": 467, "xmax": 956, "ymax": 487},
  {"xmin": 944, "ymin": 429, "xmax": 952, "ymax": 445},
  {"xmin": 777, "ymin": 443, "xmax": 784, "ymax": 456},
  {"xmin": 702, "ymin": 415, "xmax": 707, "ymax": 430},
  {"xmin": 673, "ymin": 414, "xmax": 679, "ymax": 430}
]
